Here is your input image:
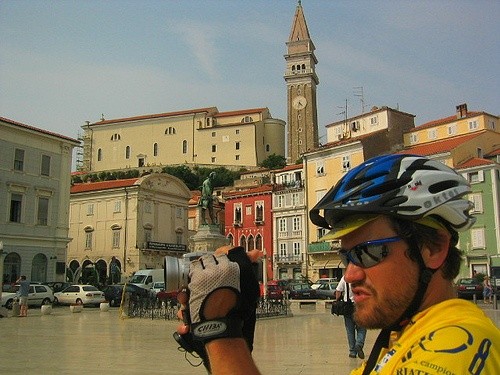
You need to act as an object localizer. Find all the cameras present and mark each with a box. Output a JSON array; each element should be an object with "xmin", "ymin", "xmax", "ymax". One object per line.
[{"xmin": 163, "ymin": 251, "xmax": 216, "ymax": 354}]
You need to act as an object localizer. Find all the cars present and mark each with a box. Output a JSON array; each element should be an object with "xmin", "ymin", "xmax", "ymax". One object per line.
[
  {"xmin": 266, "ymin": 277, "xmax": 345, "ymax": 300},
  {"xmin": 1, "ymin": 283, "xmax": 55, "ymax": 310},
  {"xmin": 454, "ymin": 278, "xmax": 485, "ymax": 300},
  {"xmin": 29, "ymin": 280, "xmax": 76, "ymax": 294},
  {"xmin": 95, "ymin": 281, "xmax": 165, "ymax": 307},
  {"xmin": 52, "ymin": 284, "xmax": 106, "ymax": 308}
]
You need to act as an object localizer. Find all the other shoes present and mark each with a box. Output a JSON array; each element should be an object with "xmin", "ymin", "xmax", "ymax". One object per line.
[
  {"xmin": 349, "ymin": 351, "xmax": 357, "ymax": 358},
  {"xmin": 490, "ymin": 301, "xmax": 493, "ymax": 303},
  {"xmin": 355, "ymin": 345, "xmax": 364, "ymax": 359},
  {"xmin": 484, "ymin": 302, "xmax": 488, "ymax": 304}
]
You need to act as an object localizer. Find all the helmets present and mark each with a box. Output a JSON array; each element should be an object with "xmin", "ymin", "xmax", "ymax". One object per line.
[{"xmin": 309, "ymin": 154, "xmax": 476, "ymax": 233}]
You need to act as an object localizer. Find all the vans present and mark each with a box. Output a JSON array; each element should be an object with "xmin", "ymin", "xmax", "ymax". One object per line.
[{"xmin": 128, "ymin": 269, "xmax": 164, "ymax": 292}]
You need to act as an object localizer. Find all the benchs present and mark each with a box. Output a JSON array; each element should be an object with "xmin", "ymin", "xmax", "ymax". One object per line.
[{"xmin": 289, "ymin": 299, "xmax": 336, "ymax": 311}]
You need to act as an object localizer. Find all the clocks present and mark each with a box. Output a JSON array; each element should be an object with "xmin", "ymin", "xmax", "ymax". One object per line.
[{"xmin": 292, "ymin": 95, "xmax": 307, "ymax": 111}]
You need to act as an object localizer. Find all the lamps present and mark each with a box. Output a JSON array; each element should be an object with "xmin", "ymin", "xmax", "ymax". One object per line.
[{"xmin": 126, "ymin": 258, "xmax": 130, "ymax": 263}]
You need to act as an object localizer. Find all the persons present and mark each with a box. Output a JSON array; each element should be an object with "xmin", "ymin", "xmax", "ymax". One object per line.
[
  {"xmin": 199, "ymin": 171, "xmax": 220, "ymax": 225},
  {"xmin": 335, "ymin": 276, "xmax": 367, "ymax": 359},
  {"xmin": 177, "ymin": 154, "xmax": 500, "ymax": 375},
  {"xmin": 16, "ymin": 275, "xmax": 30, "ymax": 317},
  {"xmin": 483, "ymin": 276, "xmax": 493, "ymax": 303}
]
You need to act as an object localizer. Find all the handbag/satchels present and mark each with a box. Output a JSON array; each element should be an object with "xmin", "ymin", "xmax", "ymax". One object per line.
[{"xmin": 331, "ymin": 301, "xmax": 353, "ymax": 315}]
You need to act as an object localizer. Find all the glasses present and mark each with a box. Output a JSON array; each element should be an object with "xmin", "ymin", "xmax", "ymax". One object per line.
[{"xmin": 338, "ymin": 232, "xmax": 415, "ymax": 268}]
